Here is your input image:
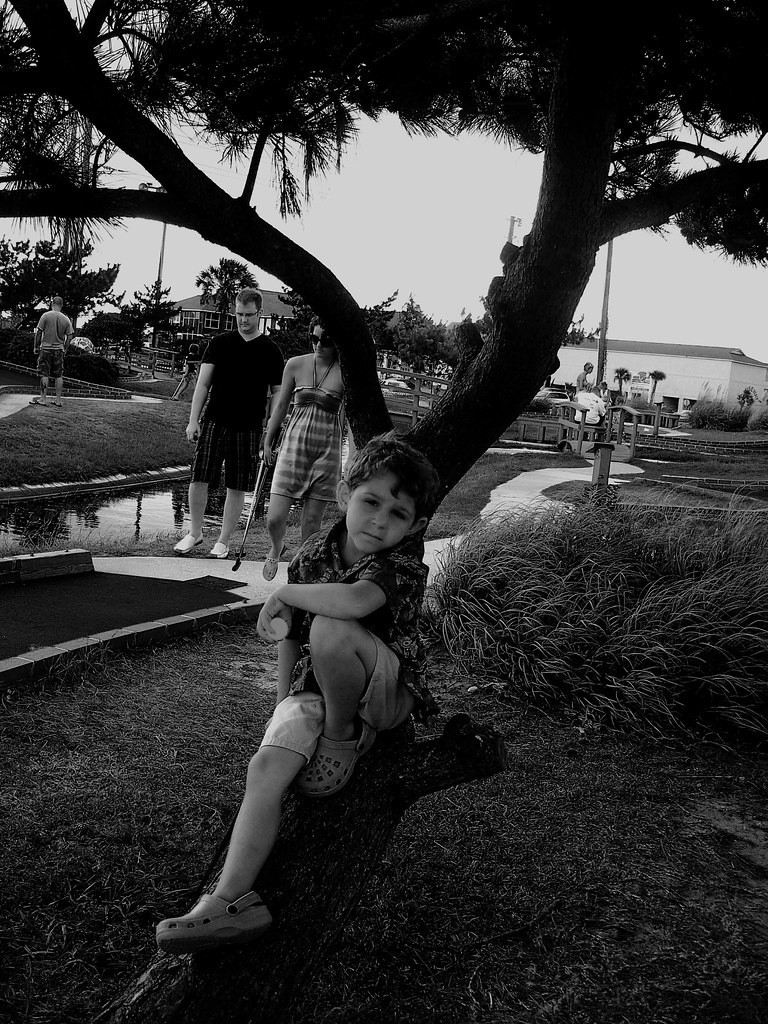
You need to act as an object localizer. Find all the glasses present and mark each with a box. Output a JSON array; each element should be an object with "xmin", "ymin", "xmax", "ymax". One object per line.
[
  {"xmin": 310, "ymin": 333, "xmax": 334, "ymax": 348},
  {"xmin": 235, "ymin": 309, "xmax": 259, "ymax": 320}
]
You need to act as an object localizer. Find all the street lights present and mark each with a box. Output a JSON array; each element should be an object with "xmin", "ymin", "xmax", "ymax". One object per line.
[{"xmin": 139, "ymin": 181, "xmax": 169, "ymax": 347}]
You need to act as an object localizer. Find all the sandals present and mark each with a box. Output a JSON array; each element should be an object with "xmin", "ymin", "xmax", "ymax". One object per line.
[
  {"xmin": 156, "ymin": 890, "xmax": 273, "ymax": 955},
  {"xmin": 296, "ymin": 715, "xmax": 377, "ymax": 798}
]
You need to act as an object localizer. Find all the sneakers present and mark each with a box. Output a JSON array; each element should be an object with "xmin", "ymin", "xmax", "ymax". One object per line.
[
  {"xmin": 173, "ymin": 530, "xmax": 204, "ymax": 554},
  {"xmin": 206, "ymin": 539, "xmax": 229, "ymax": 559}
]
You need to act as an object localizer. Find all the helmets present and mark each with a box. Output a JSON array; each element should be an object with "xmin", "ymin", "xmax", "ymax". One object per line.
[{"xmin": 584, "ymin": 362, "xmax": 594, "ymax": 371}]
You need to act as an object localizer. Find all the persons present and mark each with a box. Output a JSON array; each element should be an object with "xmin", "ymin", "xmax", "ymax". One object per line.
[
  {"xmin": 174, "ymin": 344, "xmax": 200, "ymax": 401},
  {"xmin": 29, "ymin": 296, "xmax": 73, "ymax": 407},
  {"xmin": 576, "ymin": 362, "xmax": 594, "ymax": 392},
  {"xmin": 157, "ymin": 429, "xmax": 439, "ymax": 953},
  {"xmin": 574, "ymin": 381, "xmax": 613, "ymax": 427},
  {"xmin": 262, "ymin": 315, "xmax": 358, "ymax": 581},
  {"xmin": 174, "ymin": 286, "xmax": 286, "ymax": 558}
]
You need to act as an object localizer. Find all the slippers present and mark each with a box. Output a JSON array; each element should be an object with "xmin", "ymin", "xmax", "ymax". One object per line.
[
  {"xmin": 29, "ymin": 400, "xmax": 46, "ymax": 406},
  {"xmin": 262, "ymin": 543, "xmax": 287, "ymax": 582},
  {"xmin": 173, "ymin": 397, "xmax": 179, "ymax": 401},
  {"xmin": 51, "ymin": 401, "xmax": 63, "ymax": 407}
]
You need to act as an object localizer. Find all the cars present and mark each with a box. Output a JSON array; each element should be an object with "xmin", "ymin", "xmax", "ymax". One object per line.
[{"xmin": 533, "ymin": 388, "xmax": 571, "ymax": 409}]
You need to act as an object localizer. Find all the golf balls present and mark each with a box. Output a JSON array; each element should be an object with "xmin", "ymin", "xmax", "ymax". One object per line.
[{"xmin": 265, "ymin": 617, "xmax": 289, "ymax": 641}]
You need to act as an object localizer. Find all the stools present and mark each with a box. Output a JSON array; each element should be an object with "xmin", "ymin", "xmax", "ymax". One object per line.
[{"xmin": 574, "ymin": 424, "xmax": 607, "ymax": 442}]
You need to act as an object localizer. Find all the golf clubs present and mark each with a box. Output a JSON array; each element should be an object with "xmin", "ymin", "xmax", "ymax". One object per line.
[
  {"xmin": 234, "ymin": 452, "xmax": 264, "ymax": 558},
  {"xmin": 232, "ymin": 426, "xmax": 283, "ymax": 572}
]
